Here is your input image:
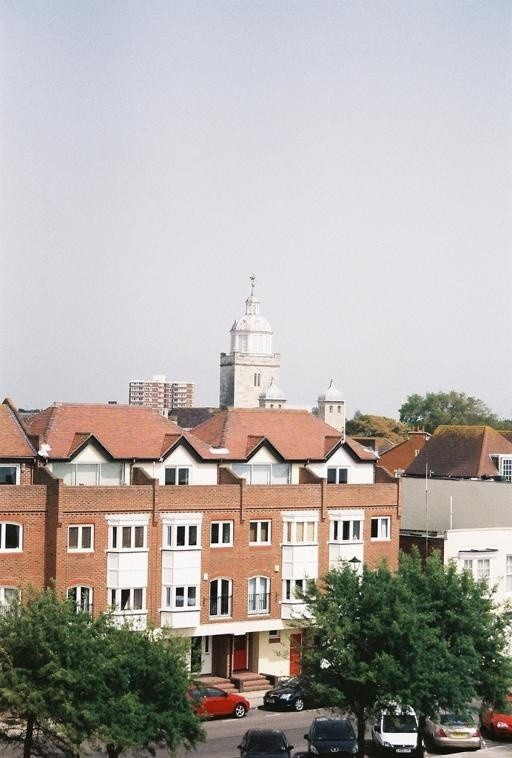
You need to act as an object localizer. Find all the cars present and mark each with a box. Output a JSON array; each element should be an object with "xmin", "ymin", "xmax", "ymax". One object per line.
[
  {"xmin": 303, "ymin": 716, "xmax": 359, "ymax": 756},
  {"xmin": 426, "ymin": 708, "xmax": 481, "ymax": 751},
  {"xmin": 262, "ymin": 677, "xmax": 328, "ymax": 710},
  {"xmin": 185, "ymin": 681, "xmax": 250, "ymax": 720},
  {"xmin": 237, "ymin": 726, "xmax": 293, "ymax": 757},
  {"xmin": 481, "ymin": 694, "xmax": 512, "ymax": 740}
]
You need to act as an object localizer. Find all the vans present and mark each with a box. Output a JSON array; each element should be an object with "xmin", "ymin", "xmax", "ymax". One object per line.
[{"xmin": 372, "ymin": 700, "xmax": 426, "ymax": 755}]
